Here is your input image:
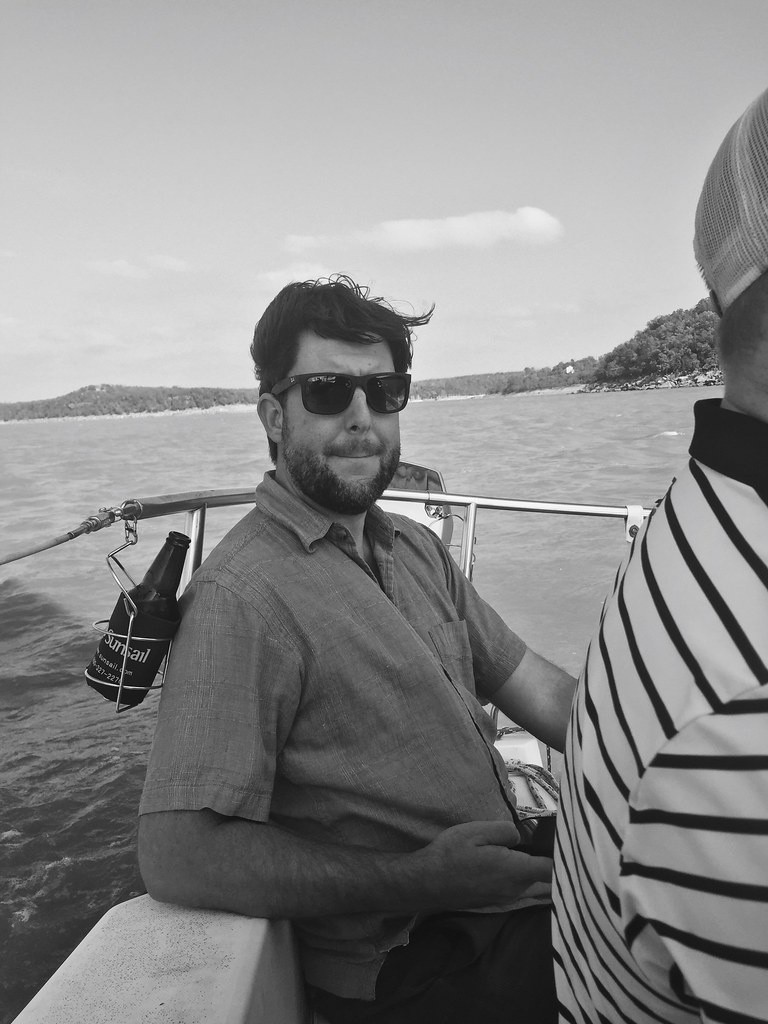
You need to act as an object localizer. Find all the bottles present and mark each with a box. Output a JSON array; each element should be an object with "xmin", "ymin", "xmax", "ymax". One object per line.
[{"xmin": 88, "ymin": 531, "xmax": 191, "ymax": 704}]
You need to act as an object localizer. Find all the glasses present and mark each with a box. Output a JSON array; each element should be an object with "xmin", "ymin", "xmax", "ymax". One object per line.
[{"xmin": 269, "ymin": 371, "xmax": 413, "ymax": 417}]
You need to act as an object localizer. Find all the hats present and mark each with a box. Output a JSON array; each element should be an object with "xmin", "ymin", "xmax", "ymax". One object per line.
[{"xmin": 693, "ymin": 87, "xmax": 768, "ymax": 318}]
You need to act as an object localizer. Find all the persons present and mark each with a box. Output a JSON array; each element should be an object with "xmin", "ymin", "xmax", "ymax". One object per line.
[
  {"xmin": 550, "ymin": 90, "xmax": 768, "ymax": 1024},
  {"xmin": 137, "ymin": 275, "xmax": 579, "ymax": 1024}
]
